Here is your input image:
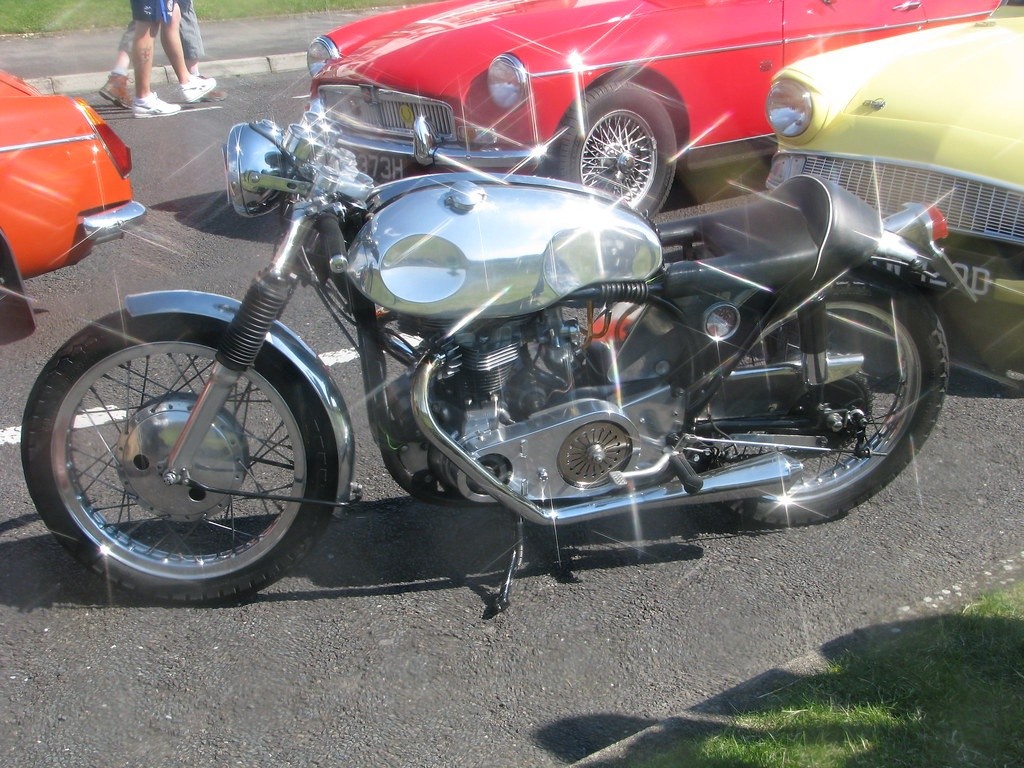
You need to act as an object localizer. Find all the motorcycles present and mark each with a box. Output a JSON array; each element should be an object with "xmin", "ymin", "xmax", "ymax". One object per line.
[{"xmin": 18, "ymin": 97, "xmax": 953, "ymax": 615}]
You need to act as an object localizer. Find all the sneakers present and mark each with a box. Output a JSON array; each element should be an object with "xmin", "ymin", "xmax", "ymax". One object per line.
[
  {"xmin": 131, "ymin": 92, "xmax": 181, "ymax": 118},
  {"xmin": 180, "ymin": 75, "xmax": 216, "ymax": 103}
]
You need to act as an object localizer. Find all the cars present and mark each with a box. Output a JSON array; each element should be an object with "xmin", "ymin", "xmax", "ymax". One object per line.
[
  {"xmin": 766, "ymin": 2, "xmax": 1024, "ymax": 394},
  {"xmin": 305, "ymin": 2, "xmax": 1007, "ymax": 217},
  {"xmin": 0, "ymin": 71, "xmax": 147, "ymax": 334}
]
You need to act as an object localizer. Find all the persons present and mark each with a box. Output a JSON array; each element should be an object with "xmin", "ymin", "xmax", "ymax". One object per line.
[
  {"xmin": 99, "ymin": 0, "xmax": 228, "ymax": 110},
  {"xmin": 128, "ymin": 0, "xmax": 216, "ymax": 119}
]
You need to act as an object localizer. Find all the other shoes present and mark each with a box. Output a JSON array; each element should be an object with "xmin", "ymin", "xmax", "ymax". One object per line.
[
  {"xmin": 196, "ymin": 74, "xmax": 228, "ymax": 102},
  {"xmin": 100, "ymin": 72, "xmax": 134, "ymax": 108}
]
